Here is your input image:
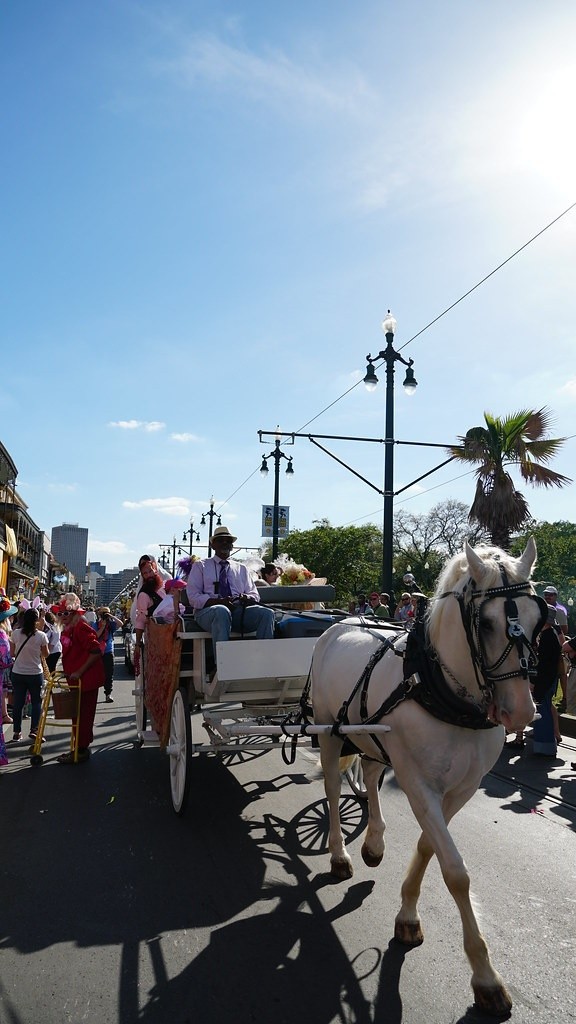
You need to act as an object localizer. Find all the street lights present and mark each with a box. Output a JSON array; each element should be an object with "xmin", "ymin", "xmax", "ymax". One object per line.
[
  {"xmin": 156, "ymin": 494, "xmax": 222, "ymax": 579},
  {"xmin": 363, "ymin": 309, "xmax": 418, "ymax": 611},
  {"xmin": 259, "ymin": 425, "xmax": 295, "ymax": 563}
]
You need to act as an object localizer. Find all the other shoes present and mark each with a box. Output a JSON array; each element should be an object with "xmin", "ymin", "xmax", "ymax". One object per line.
[
  {"xmin": 209, "ymin": 667, "xmax": 218, "ymax": 683},
  {"xmin": 507, "ymin": 738, "xmax": 524, "ymax": 749},
  {"xmin": 57, "ymin": 748, "xmax": 91, "ymax": 764},
  {"xmin": 29, "ymin": 730, "xmax": 46, "ymax": 743},
  {"xmin": 106, "ymin": 696, "xmax": 114, "ymax": 703},
  {"xmin": 555, "ymin": 699, "xmax": 566, "ymax": 712},
  {"xmin": 13, "ymin": 733, "xmax": 23, "ymax": 741},
  {"xmin": 556, "ymin": 736, "xmax": 562, "ymax": 742}
]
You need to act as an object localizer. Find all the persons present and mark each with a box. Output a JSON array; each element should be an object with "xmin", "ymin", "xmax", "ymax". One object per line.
[
  {"xmin": 532, "ymin": 585, "xmax": 576, "ymax": 757},
  {"xmin": 0, "ymin": 594, "xmax": 63, "ymax": 765},
  {"xmin": 186, "ymin": 528, "xmax": 275, "ymax": 664},
  {"xmin": 403, "ymin": 573, "xmax": 424, "ymax": 593},
  {"xmin": 133, "ymin": 555, "xmax": 187, "ymax": 677},
  {"xmin": 55, "ymin": 592, "xmax": 107, "ymax": 761},
  {"xmin": 349, "ymin": 592, "xmax": 424, "ymax": 620},
  {"xmin": 256, "ymin": 563, "xmax": 280, "ymax": 585},
  {"xmin": 83, "ymin": 607, "xmax": 124, "ymax": 701}
]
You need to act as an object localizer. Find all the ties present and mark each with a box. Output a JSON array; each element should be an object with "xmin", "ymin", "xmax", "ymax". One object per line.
[{"xmin": 219, "ymin": 560, "xmax": 229, "ymax": 599}]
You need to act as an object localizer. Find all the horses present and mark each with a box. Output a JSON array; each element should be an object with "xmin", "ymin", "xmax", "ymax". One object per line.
[{"xmin": 309, "ymin": 532, "xmax": 541, "ymax": 1018}]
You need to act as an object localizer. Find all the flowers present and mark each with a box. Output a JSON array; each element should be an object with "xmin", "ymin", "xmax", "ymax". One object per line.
[{"xmin": 276, "ymin": 559, "xmax": 315, "ymax": 585}]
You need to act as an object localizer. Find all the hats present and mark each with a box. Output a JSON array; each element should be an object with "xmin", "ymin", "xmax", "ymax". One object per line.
[
  {"xmin": 209, "ymin": 527, "xmax": 238, "ymax": 544},
  {"xmin": 542, "ymin": 585, "xmax": 558, "ymax": 594},
  {"xmin": 52, "ymin": 592, "xmax": 86, "ymax": 616},
  {"xmin": 403, "ymin": 573, "xmax": 414, "ymax": 580},
  {"xmin": 544, "ymin": 602, "xmax": 558, "ymax": 625},
  {"xmin": 0, "ymin": 601, "xmax": 18, "ymax": 622}
]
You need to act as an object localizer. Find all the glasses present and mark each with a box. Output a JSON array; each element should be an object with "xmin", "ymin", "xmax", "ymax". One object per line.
[
  {"xmin": 370, "ymin": 598, "xmax": 376, "ymax": 601},
  {"xmin": 56, "ymin": 611, "xmax": 73, "ymax": 617},
  {"xmin": 402, "ymin": 598, "xmax": 409, "ymax": 601},
  {"xmin": 404, "ymin": 581, "xmax": 411, "ymax": 584}
]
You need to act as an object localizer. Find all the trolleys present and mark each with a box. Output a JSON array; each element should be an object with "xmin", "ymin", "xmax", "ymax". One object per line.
[{"xmin": 29, "ymin": 670, "xmax": 82, "ymax": 768}]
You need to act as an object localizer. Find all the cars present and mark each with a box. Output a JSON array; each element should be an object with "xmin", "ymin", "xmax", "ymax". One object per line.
[{"xmin": 124, "ymin": 627, "xmax": 144, "ymax": 674}]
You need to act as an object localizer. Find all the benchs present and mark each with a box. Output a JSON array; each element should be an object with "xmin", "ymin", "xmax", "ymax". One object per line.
[{"xmin": 175, "ymin": 585, "xmax": 336, "ymax": 639}]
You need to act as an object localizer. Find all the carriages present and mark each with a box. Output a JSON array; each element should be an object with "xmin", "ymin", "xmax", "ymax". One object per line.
[{"xmin": 130, "ymin": 532, "xmax": 547, "ymax": 1017}]
